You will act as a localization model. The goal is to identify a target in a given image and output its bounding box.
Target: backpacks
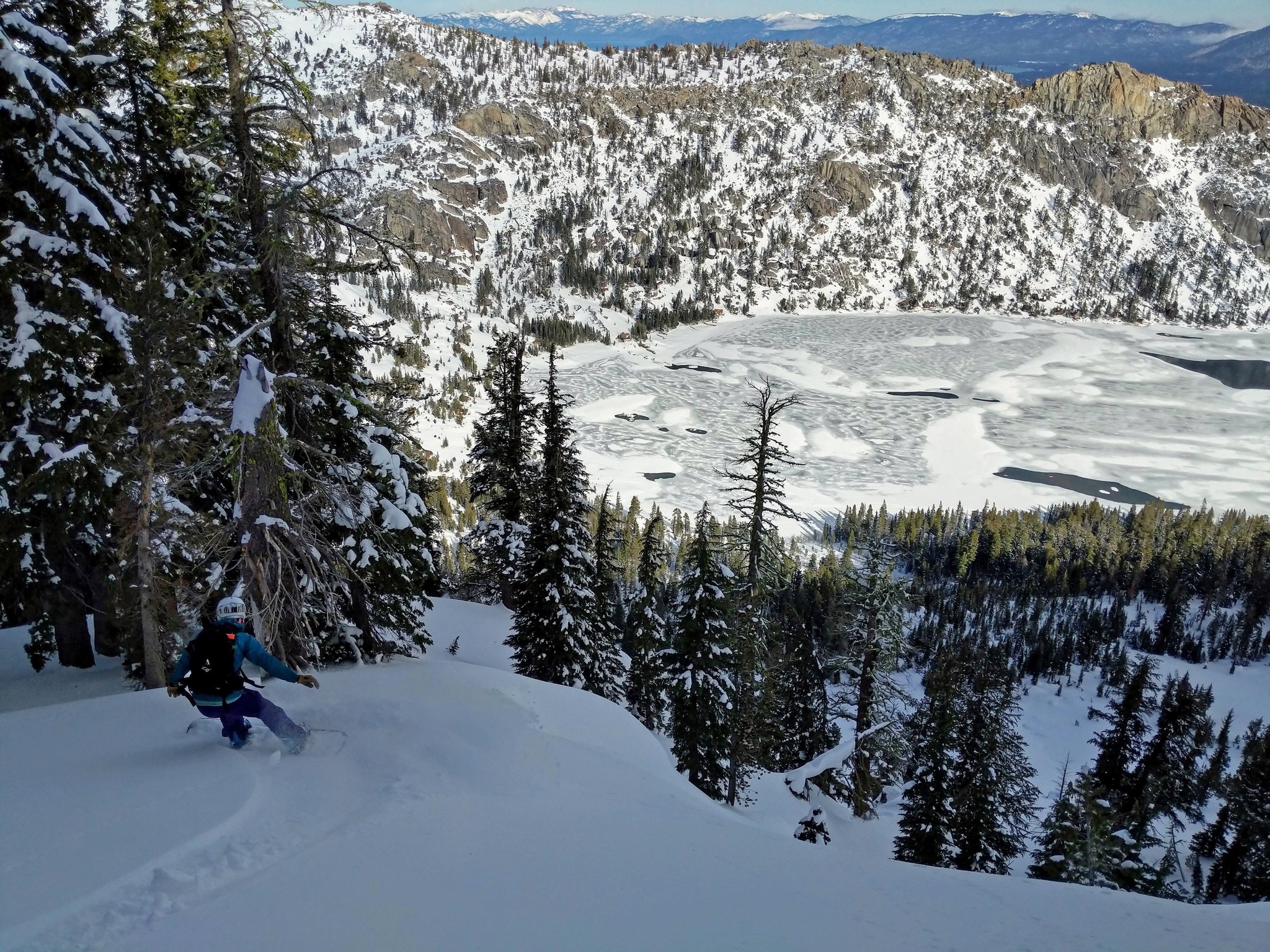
[188,624,241,697]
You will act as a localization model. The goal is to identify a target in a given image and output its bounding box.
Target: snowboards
[186,718,348,758]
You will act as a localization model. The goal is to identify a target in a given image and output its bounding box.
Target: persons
[167,598,319,754]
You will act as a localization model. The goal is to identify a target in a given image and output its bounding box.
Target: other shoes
[231,719,251,748]
[288,723,311,754]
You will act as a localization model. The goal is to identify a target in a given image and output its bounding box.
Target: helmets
[217,597,246,620]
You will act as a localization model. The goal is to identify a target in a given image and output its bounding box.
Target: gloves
[298,674,319,689]
[166,685,180,698]
